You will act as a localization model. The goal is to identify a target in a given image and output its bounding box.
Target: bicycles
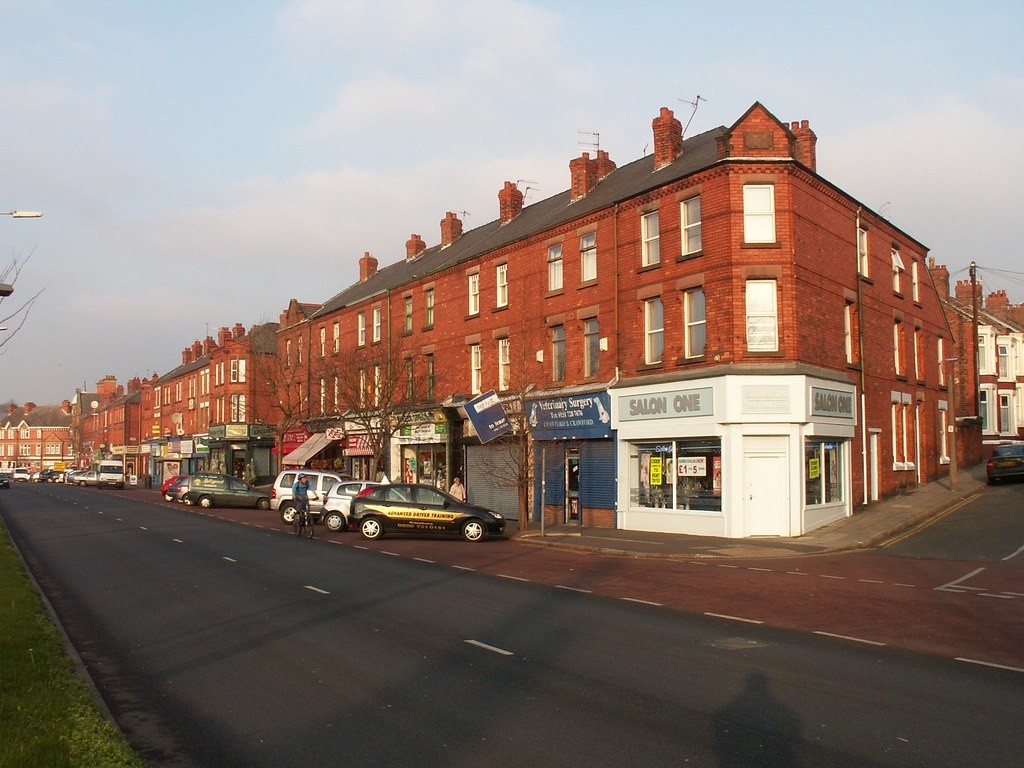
[293,496,316,539]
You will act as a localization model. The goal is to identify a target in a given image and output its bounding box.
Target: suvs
[67,470,86,485]
[270,469,358,526]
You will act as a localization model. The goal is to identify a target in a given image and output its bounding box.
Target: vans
[97,460,124,490]
[14,468,30,482]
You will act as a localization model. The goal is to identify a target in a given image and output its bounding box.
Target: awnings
[282,432,338,466]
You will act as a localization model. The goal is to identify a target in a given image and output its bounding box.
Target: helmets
[298,473,306,479]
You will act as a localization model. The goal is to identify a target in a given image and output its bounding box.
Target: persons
[292,473,319,529]
[448,477,467,503]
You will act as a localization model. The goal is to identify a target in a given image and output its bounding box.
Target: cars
[321,481,410,533]
[187,471,270,510]
[69,471,97,487]
[32,469,76,483]
[347,483,504,541]
[161,474,189,502]
[167,478,195,506]
[986,442,1024,486]
[0,474,10,489]
